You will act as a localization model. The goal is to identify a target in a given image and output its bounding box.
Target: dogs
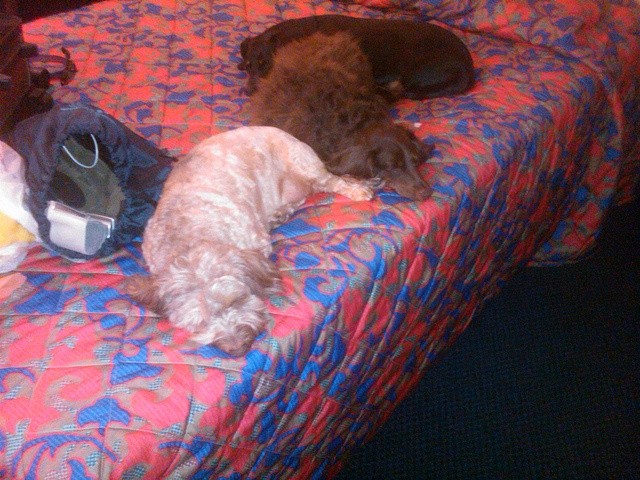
[124,126,376,357]
[250,30,435,200]
[238,14,473,100]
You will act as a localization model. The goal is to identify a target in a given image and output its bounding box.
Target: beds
[2,0,638,480]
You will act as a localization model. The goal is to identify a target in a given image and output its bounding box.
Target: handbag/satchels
[0,103,173,261]
